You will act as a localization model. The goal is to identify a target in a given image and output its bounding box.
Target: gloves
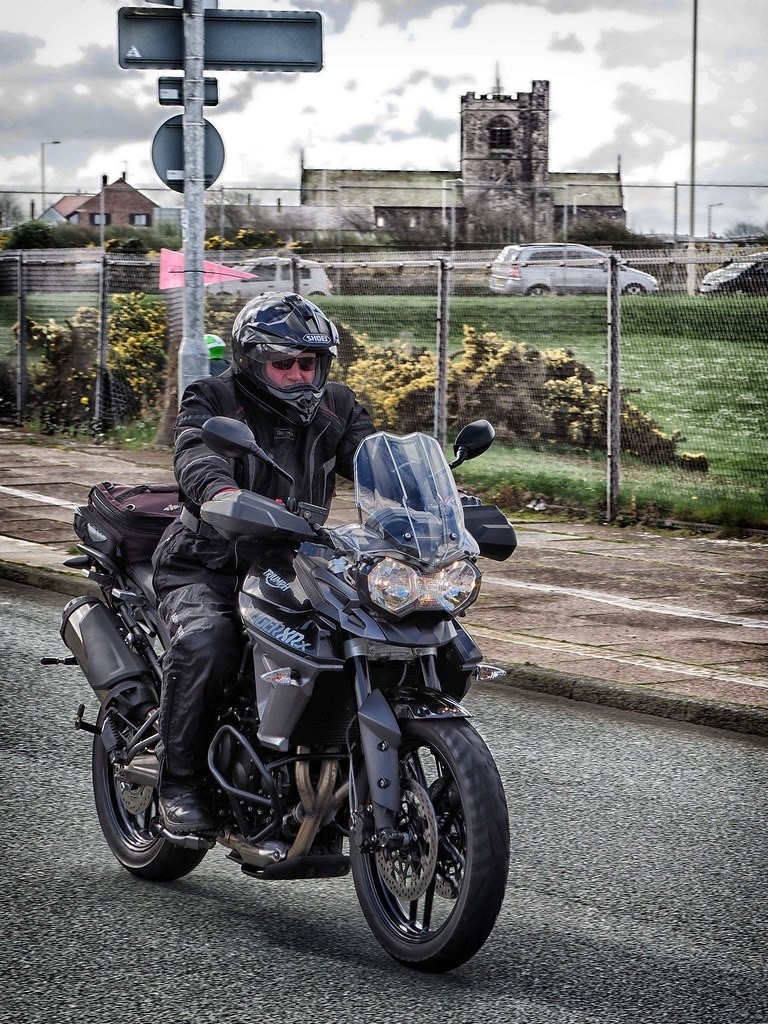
[444,492,483,508]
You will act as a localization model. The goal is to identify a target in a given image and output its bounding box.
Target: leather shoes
[158,792,215,832]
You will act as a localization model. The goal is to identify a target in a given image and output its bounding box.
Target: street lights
[37,138,63,213]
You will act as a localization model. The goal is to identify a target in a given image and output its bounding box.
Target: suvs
[488,241,660,296]
[204,257,337,299]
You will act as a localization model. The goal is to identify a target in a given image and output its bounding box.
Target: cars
[701,251,768,293]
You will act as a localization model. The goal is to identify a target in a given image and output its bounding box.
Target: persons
[150,291,484,833]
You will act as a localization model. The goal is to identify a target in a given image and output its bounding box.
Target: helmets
[231,291,339,427]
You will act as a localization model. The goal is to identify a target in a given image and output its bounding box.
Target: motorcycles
[40,419,519,961]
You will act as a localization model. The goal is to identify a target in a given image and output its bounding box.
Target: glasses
[263,351,319,371]
